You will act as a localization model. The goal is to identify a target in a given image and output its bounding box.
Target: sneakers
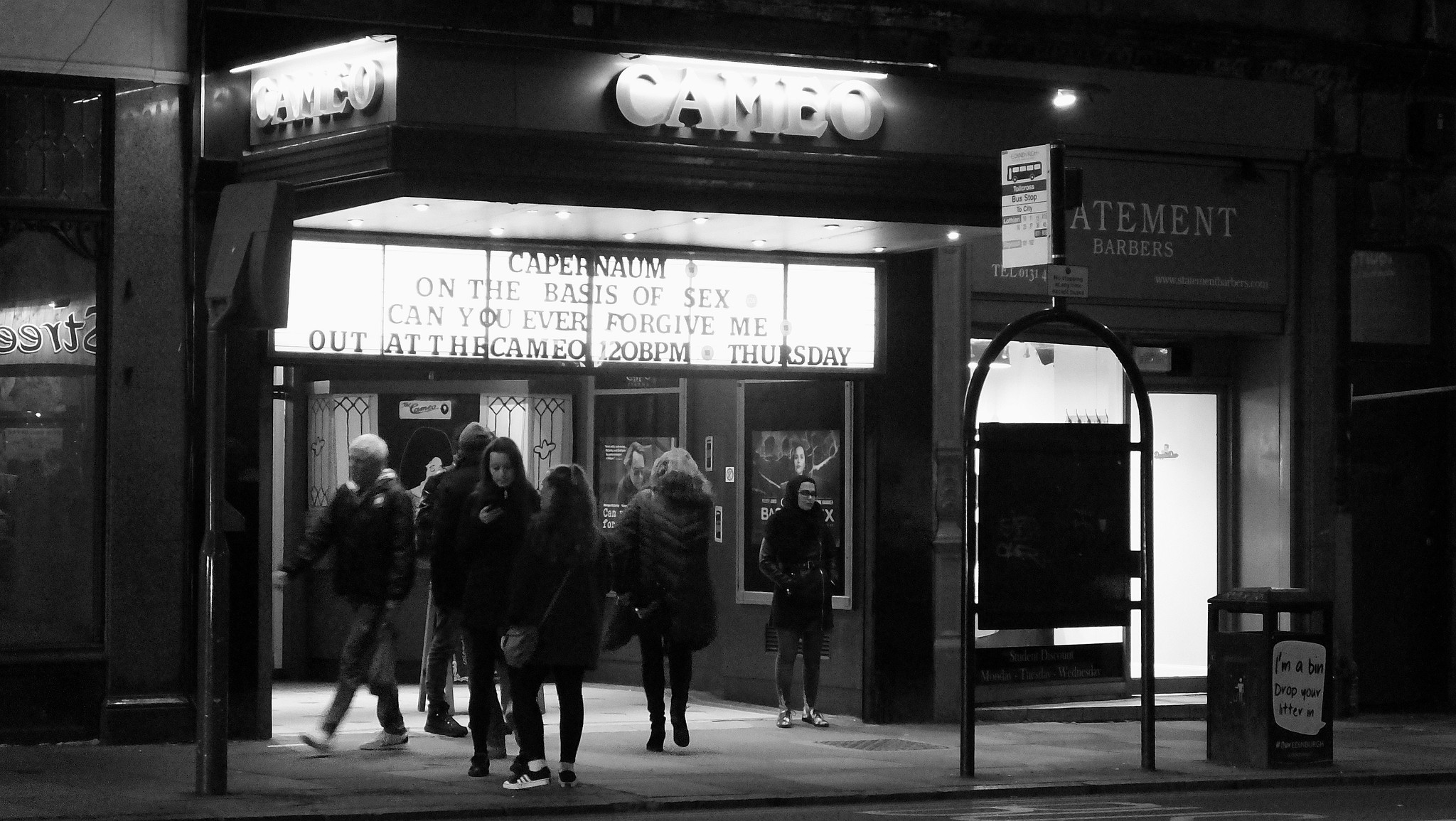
[360,729,410,750]
[558,768,577,787]
[302,729,338,755]
[424,704,468,738]
[802,708,829,728]
[776,710,792,729]
[503,766,551,789]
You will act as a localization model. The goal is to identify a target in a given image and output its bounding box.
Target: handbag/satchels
[499,625,539,667]
[599,591,639,650]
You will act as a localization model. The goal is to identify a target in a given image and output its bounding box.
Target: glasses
[798,490,818,498]
[631,466,646,475]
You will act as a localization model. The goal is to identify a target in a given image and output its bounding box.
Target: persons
[454,437,542,776]
[275,434,418,755]
[759,474,837,729]
[609,450,717,752]
[616,440,652,510]
[790,444,806,476]
[418,422,514,739]
[504,461,609,790]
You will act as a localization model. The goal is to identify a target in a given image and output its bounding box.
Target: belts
[778,560,830,571]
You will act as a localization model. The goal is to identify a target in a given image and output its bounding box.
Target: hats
[458,421,491,452]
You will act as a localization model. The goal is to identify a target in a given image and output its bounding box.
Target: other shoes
[468,755,490,777]
[510,750,533,773]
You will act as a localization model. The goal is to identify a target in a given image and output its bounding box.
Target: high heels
[671,707,690,747]
[646,725,666,752]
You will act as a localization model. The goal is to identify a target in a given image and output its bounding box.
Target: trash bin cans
[1205,586,1334,771]
